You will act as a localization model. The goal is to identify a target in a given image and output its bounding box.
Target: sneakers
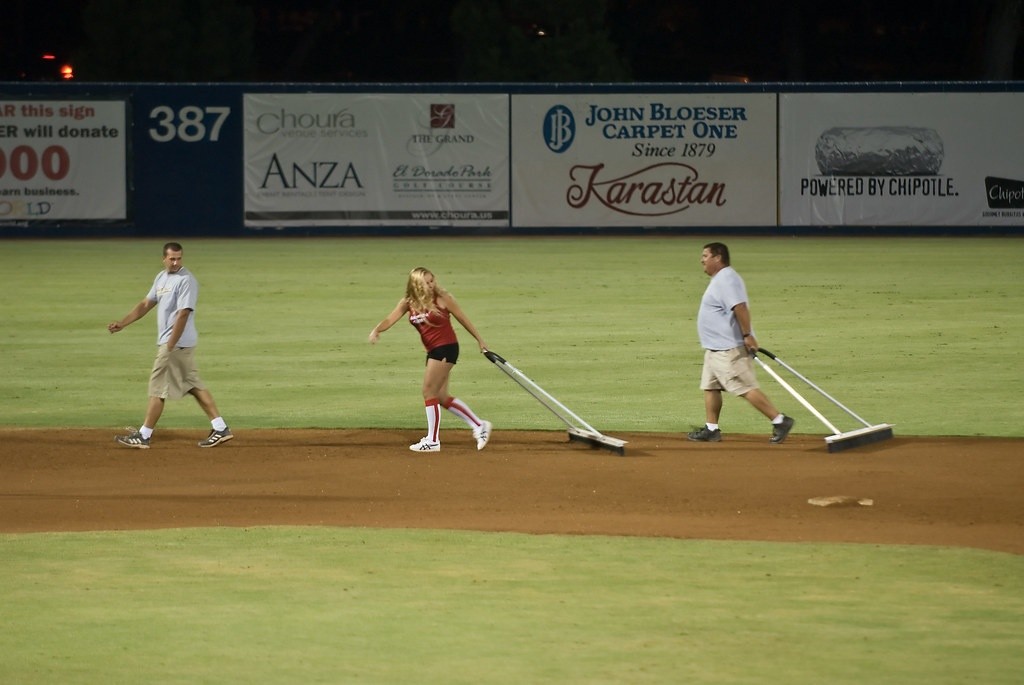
[410,436,440,452]
[473,421,492,451]
[117,426,151,449]
[198,426,233,447]
[687,424,722,442]
[769,413,794,444]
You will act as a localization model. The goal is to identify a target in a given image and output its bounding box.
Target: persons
[369,268,492,453]
[108,243,234,448]
[687,242,794,443]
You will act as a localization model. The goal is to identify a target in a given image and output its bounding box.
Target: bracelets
[743,333,751,339]
